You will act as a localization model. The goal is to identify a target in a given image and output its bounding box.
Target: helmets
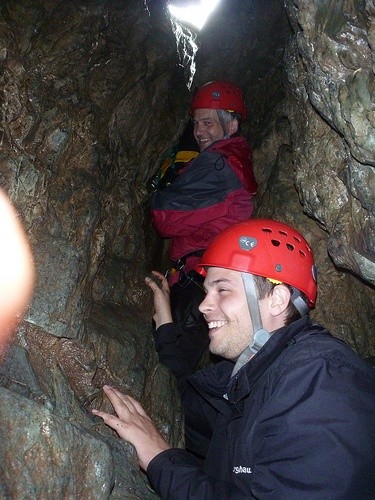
[191,80,248,119]
[194,216,320,307]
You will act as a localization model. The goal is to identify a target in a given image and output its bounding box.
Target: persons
[145,78,261,465]
[91,219,375,500]
